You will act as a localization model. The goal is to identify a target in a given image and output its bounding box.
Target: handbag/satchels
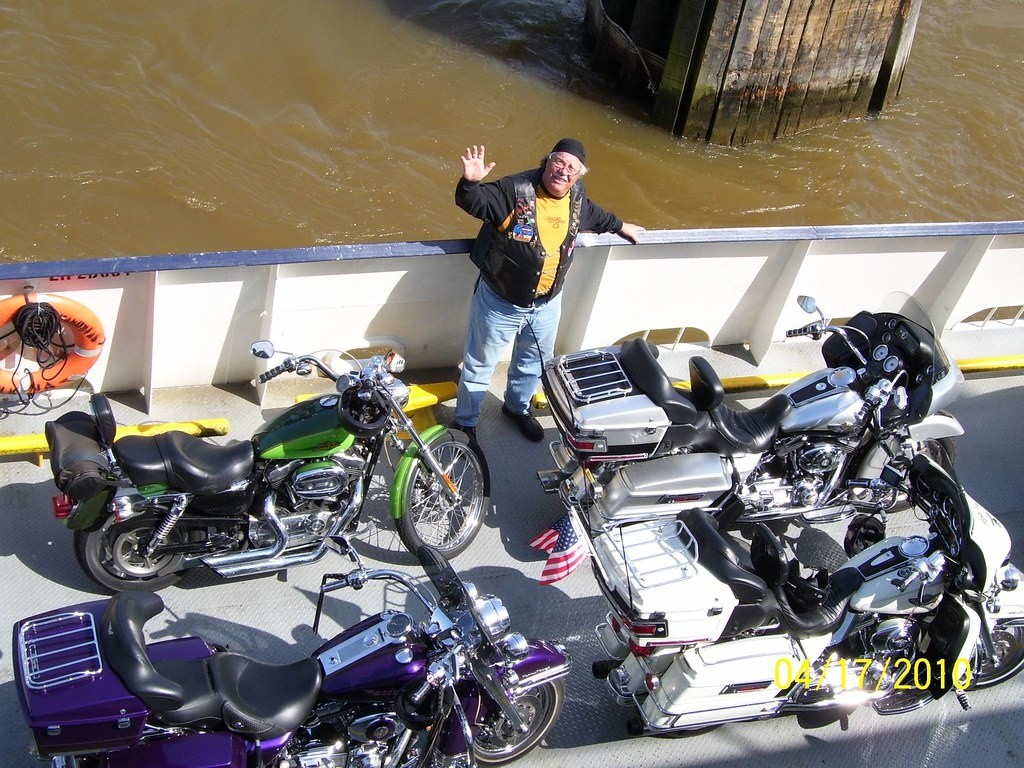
[45,411,110,499]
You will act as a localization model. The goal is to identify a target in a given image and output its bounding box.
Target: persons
[454,138,646,442]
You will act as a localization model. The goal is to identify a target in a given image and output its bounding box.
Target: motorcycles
[45,340,492,595]
[520,289,966,540]
[9,532,576,768]
[581,438,1024,738]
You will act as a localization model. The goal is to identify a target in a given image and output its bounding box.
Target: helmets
[338,380,392,438]
[393,675,454,733]
[844,514,886,558]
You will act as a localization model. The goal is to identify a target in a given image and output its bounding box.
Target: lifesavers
[0,290,107,394]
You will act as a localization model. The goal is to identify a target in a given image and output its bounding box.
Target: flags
[527,511,592,586]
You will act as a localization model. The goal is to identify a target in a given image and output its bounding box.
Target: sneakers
[453,422,476,459]
[502,402,544,442]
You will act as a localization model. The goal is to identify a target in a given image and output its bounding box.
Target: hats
[551,138,585,165]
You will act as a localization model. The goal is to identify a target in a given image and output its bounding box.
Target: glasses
[548,156,580,176]
